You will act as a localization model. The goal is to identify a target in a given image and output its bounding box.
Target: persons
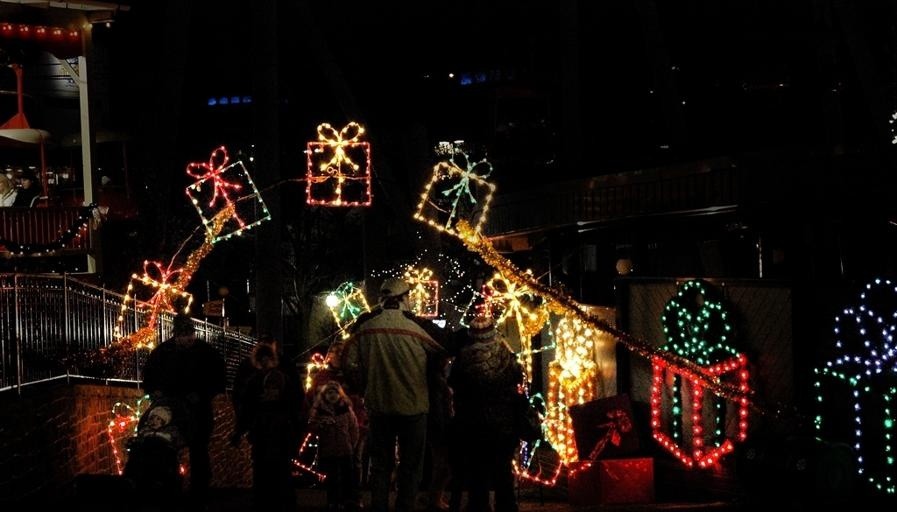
[13,169,39,209]
[121,268,542,510]
[0,174,18,209]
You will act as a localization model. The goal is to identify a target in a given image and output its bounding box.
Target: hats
[169,313,200,334]
[148,404,174,427]
[379,276,412,298]
[467,316,495,340]
[321,379,344,399]
